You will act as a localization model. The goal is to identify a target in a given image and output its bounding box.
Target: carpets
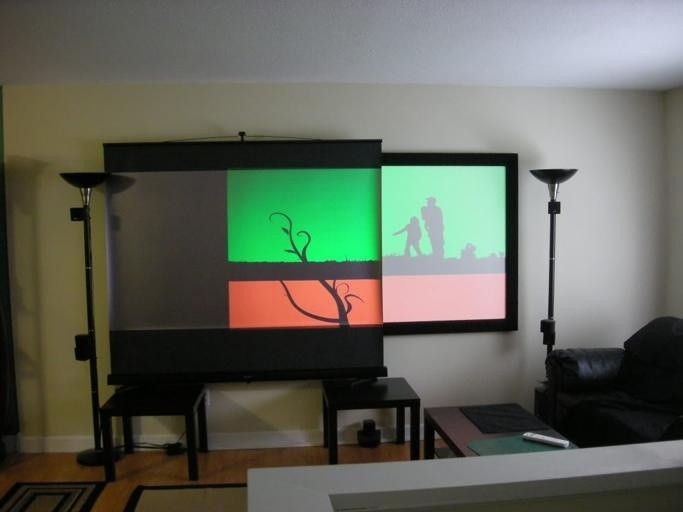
[0,478,246,512]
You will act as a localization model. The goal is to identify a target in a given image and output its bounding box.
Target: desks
[94,379,211,484]
[321,375,420,466]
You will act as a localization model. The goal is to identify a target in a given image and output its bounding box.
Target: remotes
[522,432,569,448]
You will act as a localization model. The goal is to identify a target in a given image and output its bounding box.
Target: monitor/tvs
[217,152,519,336]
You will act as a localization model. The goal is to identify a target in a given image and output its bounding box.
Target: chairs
[532,316,681,443]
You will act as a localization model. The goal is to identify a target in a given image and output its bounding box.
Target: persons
[393,216,421,256]
[421,197,444,257]
